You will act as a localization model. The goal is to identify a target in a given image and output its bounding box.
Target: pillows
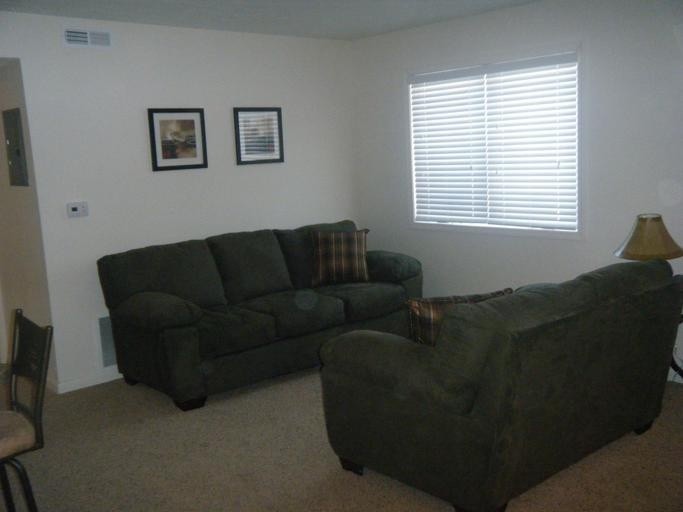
[396,288,512,347]
[311,228,369,288]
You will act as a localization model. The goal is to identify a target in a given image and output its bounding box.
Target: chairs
[0,309,53,511]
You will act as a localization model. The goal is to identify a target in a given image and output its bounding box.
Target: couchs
[320,262,682,511]
[95,219,422,410]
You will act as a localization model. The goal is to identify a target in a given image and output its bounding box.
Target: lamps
[613,213,682,261]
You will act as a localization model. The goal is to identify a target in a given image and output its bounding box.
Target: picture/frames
[147,108,208,171]
[233,107,284,166]
